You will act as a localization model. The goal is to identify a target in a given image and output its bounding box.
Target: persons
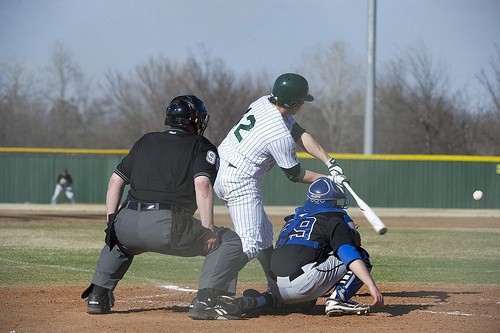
[50,168,76,207]
[80,94,245,321]
[211,73,353,306]
[235,176,385,319]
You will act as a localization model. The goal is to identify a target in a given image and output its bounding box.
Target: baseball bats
[343,180,387,235]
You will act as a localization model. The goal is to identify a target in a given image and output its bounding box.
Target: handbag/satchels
[171,203,211,250]
[104,213,117,252]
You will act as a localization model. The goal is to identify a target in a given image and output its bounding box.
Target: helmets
[270,73,314,110]
[164,94,210,136]
[307,177,349,209]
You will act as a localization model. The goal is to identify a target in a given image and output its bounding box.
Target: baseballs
[472,189,483,200]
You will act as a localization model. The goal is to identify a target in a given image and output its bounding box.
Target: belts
[122,199,171,211]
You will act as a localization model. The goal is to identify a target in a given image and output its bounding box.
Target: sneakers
[188,297,244,320]
[324,298,370,317]
[217,295,257,314]
[86,292,110,313]
